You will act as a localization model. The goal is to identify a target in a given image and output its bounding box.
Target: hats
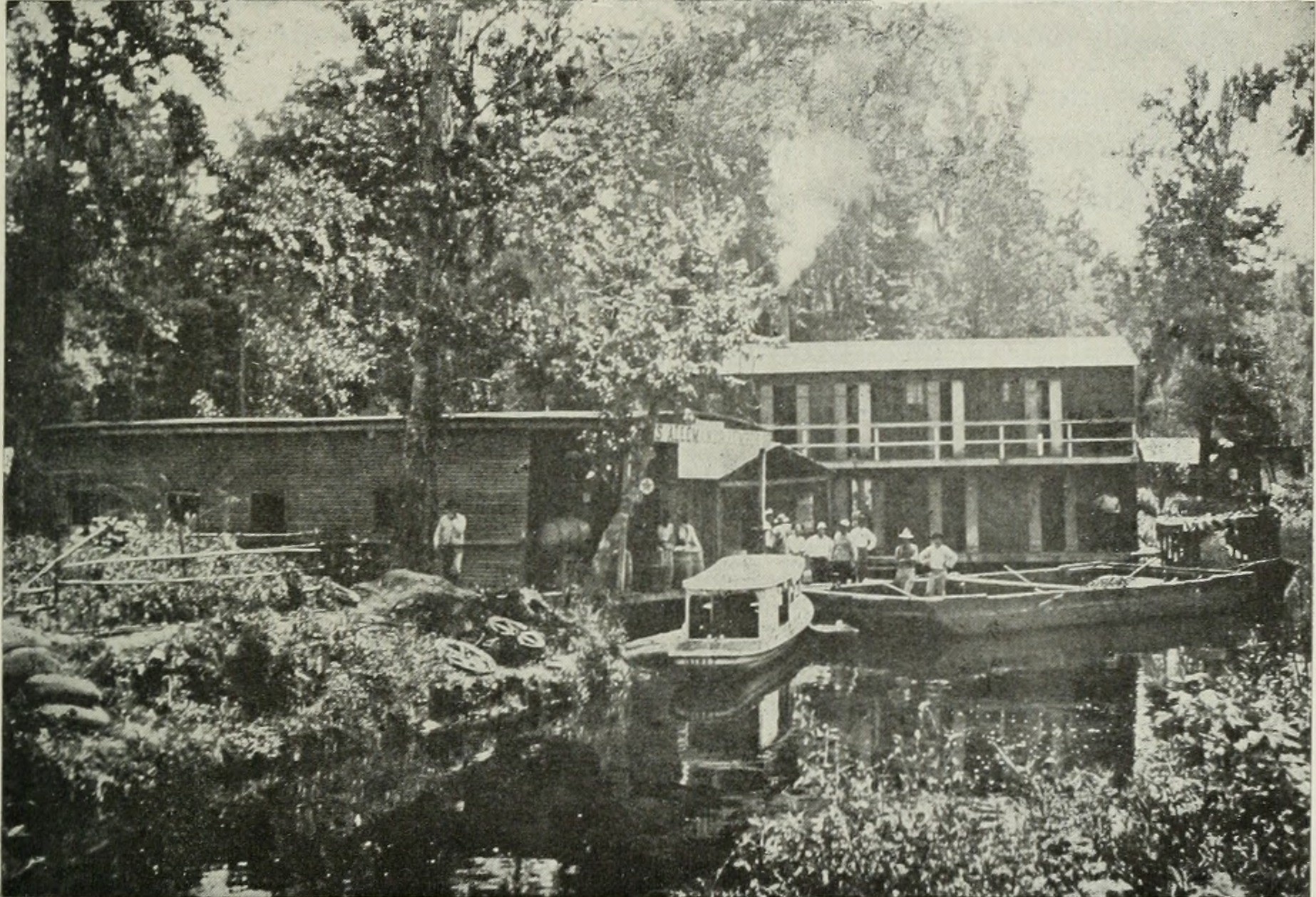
[817,521,827,530]
[898,526,914,539]
[444,499,460,509]
[774,513,789,524]
[840,518,850,527]
[927,531,942,539]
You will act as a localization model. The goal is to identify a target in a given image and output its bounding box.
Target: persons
[764,508,958,596]
[1092,486,1122,550]
[657,511,704,580]
[432,498,468,585]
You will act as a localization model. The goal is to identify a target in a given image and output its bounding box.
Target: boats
[616,551,816,684]
[794,554,1260,638]
